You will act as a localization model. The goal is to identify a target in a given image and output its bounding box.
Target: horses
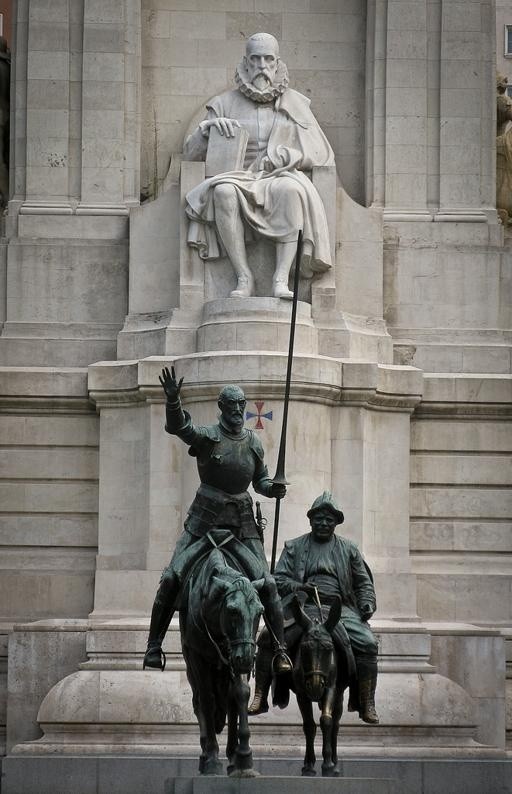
[289,593,347,777]
[180,550,266,778]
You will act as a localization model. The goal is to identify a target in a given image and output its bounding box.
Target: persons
[140,360,295,676]
[180,31,332,304]
[247,490,386,731]
[490,71,511,230]
